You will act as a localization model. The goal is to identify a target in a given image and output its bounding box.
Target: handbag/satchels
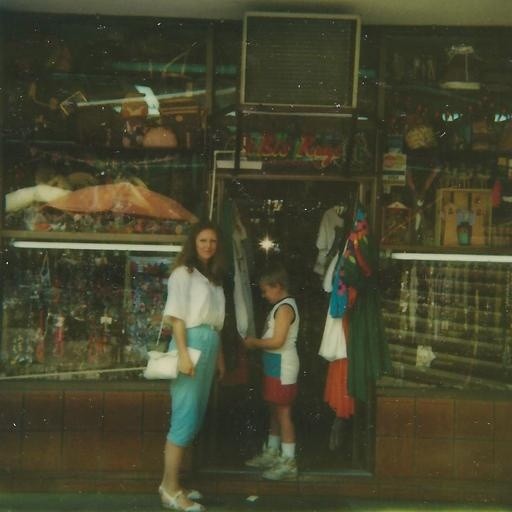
[142,349,180,381]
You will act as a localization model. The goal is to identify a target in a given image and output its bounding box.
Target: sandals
[161,490,208,511]
[159,484,204,501]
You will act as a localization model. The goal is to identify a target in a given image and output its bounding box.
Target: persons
[243,262,299,481]
[156,217,227,511]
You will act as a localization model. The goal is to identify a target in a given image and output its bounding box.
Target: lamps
[144,116,177,149]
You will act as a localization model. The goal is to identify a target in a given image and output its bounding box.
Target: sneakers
[262,456,299,481]
[244,442,280,468]
